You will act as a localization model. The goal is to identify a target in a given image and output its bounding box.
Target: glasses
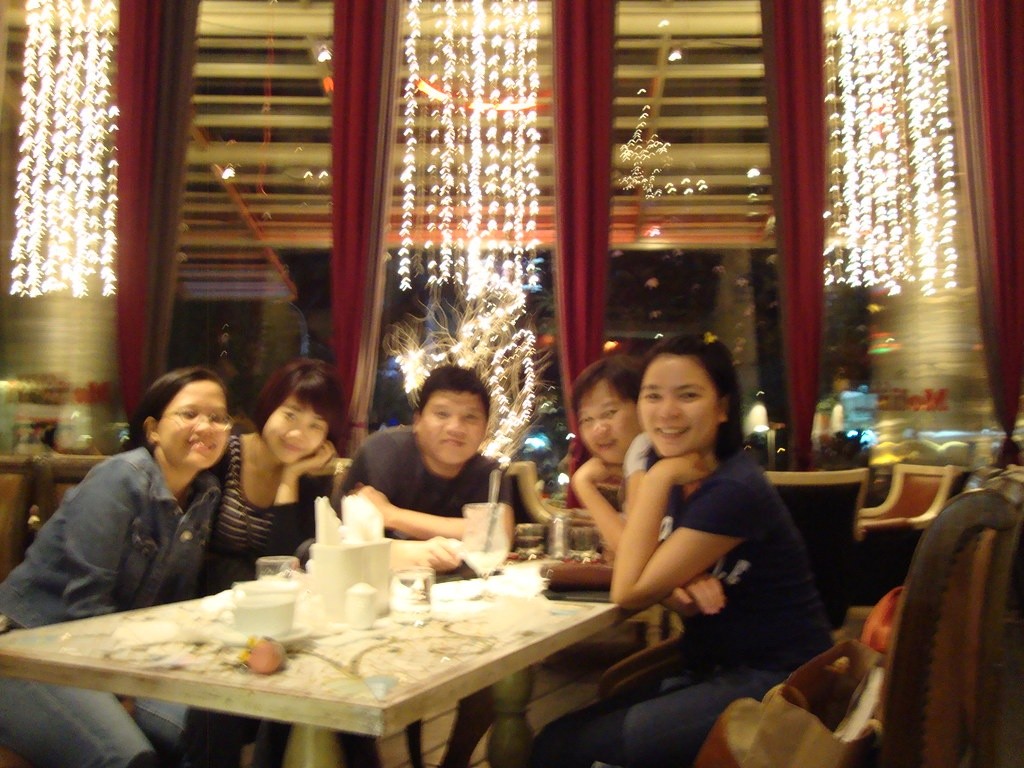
[159,407,236,431]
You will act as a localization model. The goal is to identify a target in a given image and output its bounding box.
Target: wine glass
[460,501,517,603]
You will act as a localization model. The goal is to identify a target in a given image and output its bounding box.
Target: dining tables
[0,554,659,768]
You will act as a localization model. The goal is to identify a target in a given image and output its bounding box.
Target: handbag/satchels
[859,583,905,655]
[699,635,890,768]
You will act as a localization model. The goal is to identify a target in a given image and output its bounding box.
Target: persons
[534,333,833,768]
[334,366,515,768]
[0,362,340,768]
[570,357,640,554]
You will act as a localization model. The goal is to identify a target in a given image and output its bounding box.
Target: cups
[517,522,545,560]
[254,555,301,597]
[229,596,299,638]
[563,518,599,565]
[389,565,436,627]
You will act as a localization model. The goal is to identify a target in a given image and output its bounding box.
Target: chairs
[0,451,1024,768]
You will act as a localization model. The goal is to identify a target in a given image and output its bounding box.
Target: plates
[210,625,312,650]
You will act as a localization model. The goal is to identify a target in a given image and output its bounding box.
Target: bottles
[346,582,379,631]
